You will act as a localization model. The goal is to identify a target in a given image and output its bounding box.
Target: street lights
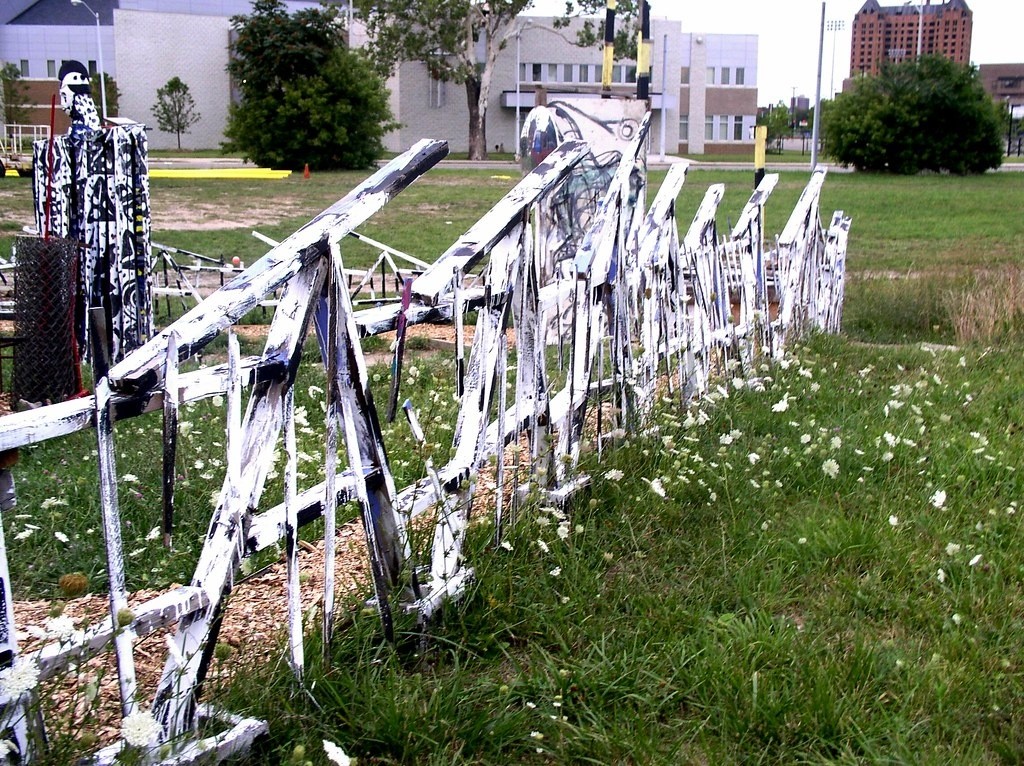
[515,18,533,162]
[70,0,108,134]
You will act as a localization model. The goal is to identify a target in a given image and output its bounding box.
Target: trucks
[0,123,53,177]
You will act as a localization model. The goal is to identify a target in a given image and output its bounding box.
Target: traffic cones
[303,163,311,179]
